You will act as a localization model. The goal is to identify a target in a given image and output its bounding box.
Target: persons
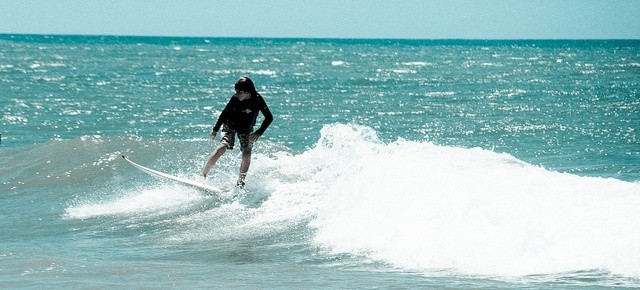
[201,76,273,188]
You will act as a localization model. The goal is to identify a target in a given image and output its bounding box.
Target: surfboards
[121,153,223,201]
